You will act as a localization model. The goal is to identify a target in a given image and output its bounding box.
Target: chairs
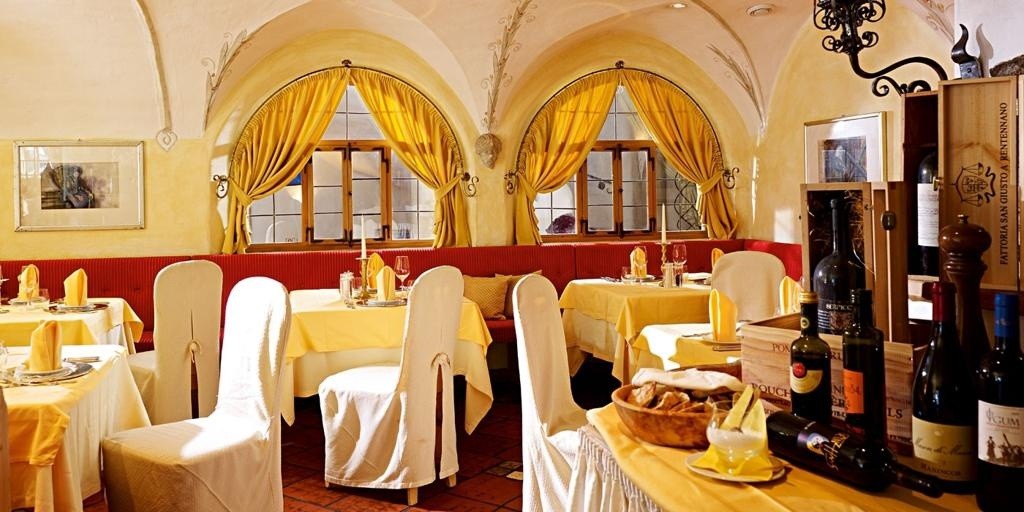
[711,251,785,327]
[97,274,290,512]
[127,260,221,424]
[266,222,298,242]
[318,263,465,505]
[512,273,587,512]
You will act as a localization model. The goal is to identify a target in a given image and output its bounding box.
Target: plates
[3,364,94,383]
[9,297,43,304]
[46,302,107,315]
[686,448,786,481]
[356,297,409,308]
[700,334,746,346]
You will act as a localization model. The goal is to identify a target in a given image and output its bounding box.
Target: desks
[281,289,495,435]
[0,296,145,354]
[0,344,152,512]
[575,397,1024,512]
[558,271,710,384]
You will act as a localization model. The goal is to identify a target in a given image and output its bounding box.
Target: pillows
[461,274,511,319]
[495,270,542,319]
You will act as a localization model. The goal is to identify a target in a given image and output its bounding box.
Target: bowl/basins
[609,382,728,449]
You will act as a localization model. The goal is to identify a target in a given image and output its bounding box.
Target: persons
[63,166,93,208]
[986,433,1024,466]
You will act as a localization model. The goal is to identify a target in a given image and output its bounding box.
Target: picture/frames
[13,140,143,232]
[804,112,887,184]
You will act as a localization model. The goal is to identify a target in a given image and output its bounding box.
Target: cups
[37,289,53,305]
[672,244,688,266]
[621,266,637,284]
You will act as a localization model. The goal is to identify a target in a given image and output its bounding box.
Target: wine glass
[633,245,649,281]
[394,255,411,296]
[19,265,41,311]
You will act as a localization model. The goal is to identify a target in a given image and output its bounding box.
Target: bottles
[909,139,939,277]
[981,289,1023,511]
[675,267,684,286]
[339,271,358,300]
[763,408,942,499]
[787,288,834,425]
[908,277,979,490]
[840,286,891,434]
[811,198,866,334]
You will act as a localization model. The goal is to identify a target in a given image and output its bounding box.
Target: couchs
[13,238,804,391]
